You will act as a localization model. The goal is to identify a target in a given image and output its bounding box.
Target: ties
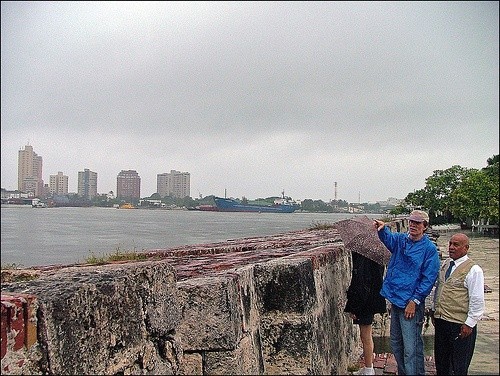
[445,261,455,281]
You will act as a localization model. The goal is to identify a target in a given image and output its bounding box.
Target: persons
[430,233,484,376]
[344,252,387,376]
[373,210,439,376]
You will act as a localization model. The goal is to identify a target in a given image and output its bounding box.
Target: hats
[406,210,429,223]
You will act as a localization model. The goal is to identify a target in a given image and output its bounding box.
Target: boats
[121,203,136,209]
[213,196,296,213]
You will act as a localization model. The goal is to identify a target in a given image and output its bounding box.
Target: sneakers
[353,363,375,375]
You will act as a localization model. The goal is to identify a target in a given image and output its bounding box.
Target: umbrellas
[333,214,392,267]
